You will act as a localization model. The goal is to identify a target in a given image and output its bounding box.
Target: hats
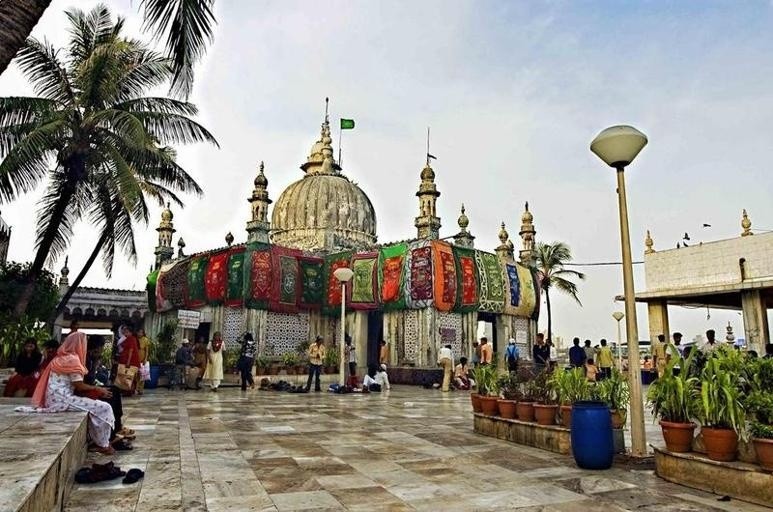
[180,339,189,343]
[509,338,515,343]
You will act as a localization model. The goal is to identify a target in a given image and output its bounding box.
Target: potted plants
[199,343,355,375]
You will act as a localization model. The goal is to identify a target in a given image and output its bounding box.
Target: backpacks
[507,345,516,367]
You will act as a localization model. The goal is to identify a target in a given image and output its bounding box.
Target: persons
[176,339,206,370]
[533,333,558,371]
[437,337,492,393]
[202,332,226,392]
[569,338,615,382]
[237,331,255,390]
[653,330,772,380]
[644,356,651,368]
[345,336,356,376]
[363,364,391,392]
[73,333,134,445]
[3,321,82,398]
[380,340,387,364]
[14,331,116,456]
[304,336,326,391]
[504,339,519,374]
[117,326,149,397]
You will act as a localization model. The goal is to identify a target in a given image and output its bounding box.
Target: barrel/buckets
[570,400,614,470]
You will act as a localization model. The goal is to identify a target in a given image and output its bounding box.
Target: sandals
[76,427,143,485]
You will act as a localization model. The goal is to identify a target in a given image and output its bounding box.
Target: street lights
[610,310,624,375]
[588,123,649,457]
[332,267,353,389]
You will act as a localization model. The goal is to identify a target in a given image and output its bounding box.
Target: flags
[340,118,354,129]
